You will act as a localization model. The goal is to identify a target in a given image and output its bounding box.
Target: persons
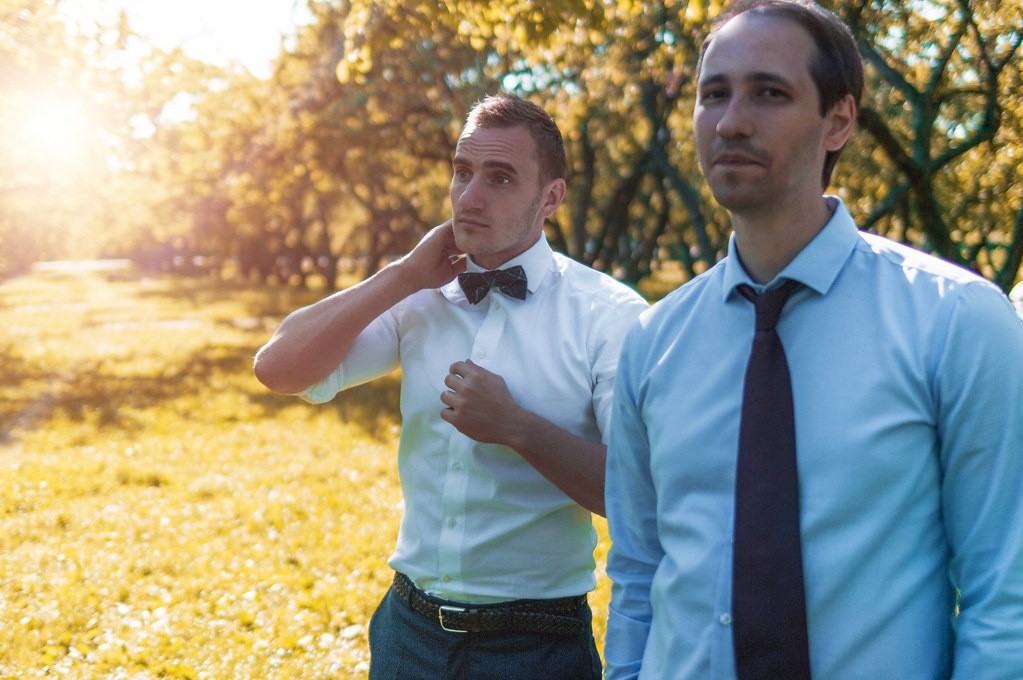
[605,0,1023,680]
[252,91,649,680]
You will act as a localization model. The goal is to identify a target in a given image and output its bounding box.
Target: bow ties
[457,265,528,304]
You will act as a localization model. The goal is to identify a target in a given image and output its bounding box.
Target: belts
[392,571,587,637]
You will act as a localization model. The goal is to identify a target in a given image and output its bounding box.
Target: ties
[737,279,812,680]
